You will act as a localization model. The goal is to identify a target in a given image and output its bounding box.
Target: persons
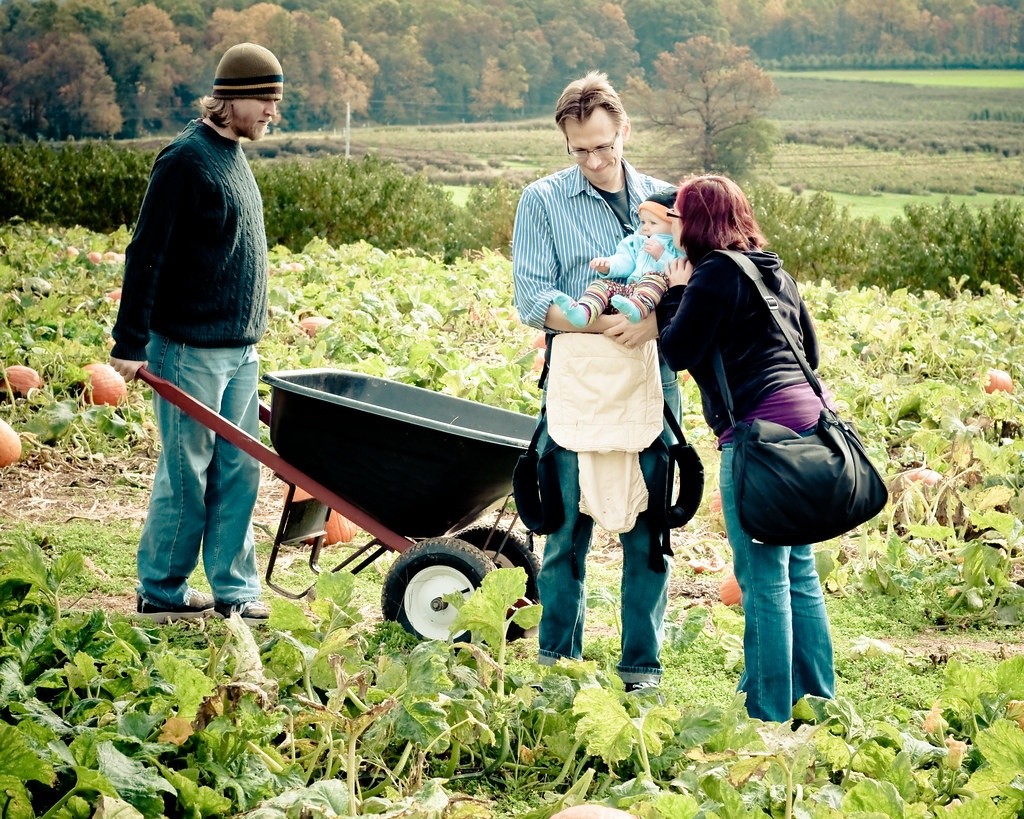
[550,186,683,329]
[655,174,834,731]
[109,44,286,619]
[511,76,685,692]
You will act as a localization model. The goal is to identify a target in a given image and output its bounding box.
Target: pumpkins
[0,245,1016,609]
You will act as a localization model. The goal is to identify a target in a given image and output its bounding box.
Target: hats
[212,42,284,100]
[638,185,679,225]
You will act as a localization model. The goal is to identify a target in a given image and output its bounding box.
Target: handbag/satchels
[733,408,889,546]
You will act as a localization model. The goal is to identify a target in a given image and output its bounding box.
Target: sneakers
[625,681,666,707]
[135,587,271,620]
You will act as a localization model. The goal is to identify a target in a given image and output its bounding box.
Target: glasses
[566,128,622,159]
[666,209,684,220]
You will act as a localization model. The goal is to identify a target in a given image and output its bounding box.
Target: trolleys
[135,362,546,654]
